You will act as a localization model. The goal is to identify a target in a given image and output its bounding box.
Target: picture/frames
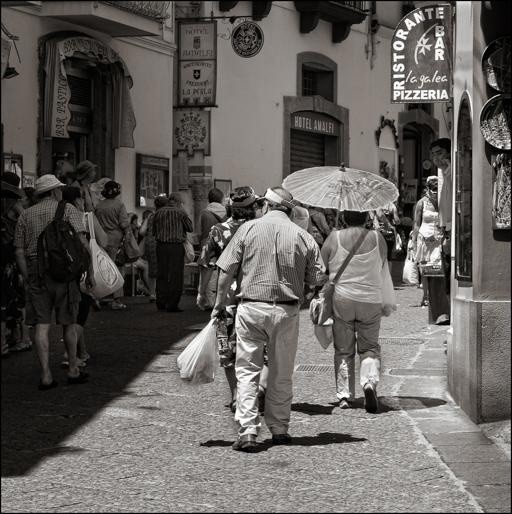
[214,178,233,198]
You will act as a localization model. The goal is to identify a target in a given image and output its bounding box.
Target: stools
[125,261,140,296]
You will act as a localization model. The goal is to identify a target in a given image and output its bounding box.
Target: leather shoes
[232,434,256,451]
[272,433,292,444]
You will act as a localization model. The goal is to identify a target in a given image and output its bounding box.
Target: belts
[241,298,298,305]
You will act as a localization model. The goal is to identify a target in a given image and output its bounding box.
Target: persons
[414,175,446,307]
[195,185,269,416]
[431,138,451,227]
[319,209,389,414]
[13,173,97,390]
[407,229,414,259]
[291,199,347,248]
[61,186,108,367]
[55,157,227,314]
[364,201,399,268]
[211,186,327,449]
[0,171,33,356]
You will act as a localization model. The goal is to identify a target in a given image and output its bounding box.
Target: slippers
[39,350,90,389]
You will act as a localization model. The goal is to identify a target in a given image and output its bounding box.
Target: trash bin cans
[423,272,450,325]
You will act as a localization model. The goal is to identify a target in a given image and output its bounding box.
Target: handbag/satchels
[403,259,420,285]
[37,218,89,284]
[80,238,124,301]
[115,231,142,267]
[309,282,335,325]
[184,240,195,263]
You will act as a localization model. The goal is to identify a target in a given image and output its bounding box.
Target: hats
[76,160,98,181]
[257,187,295,209]
[34,175,67,195]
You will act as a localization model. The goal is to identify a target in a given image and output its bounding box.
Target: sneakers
[339,398,351,408]
[111,302,127,310]
[364,384,379,413]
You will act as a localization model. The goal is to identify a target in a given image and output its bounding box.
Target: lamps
[2,33,22,79]
